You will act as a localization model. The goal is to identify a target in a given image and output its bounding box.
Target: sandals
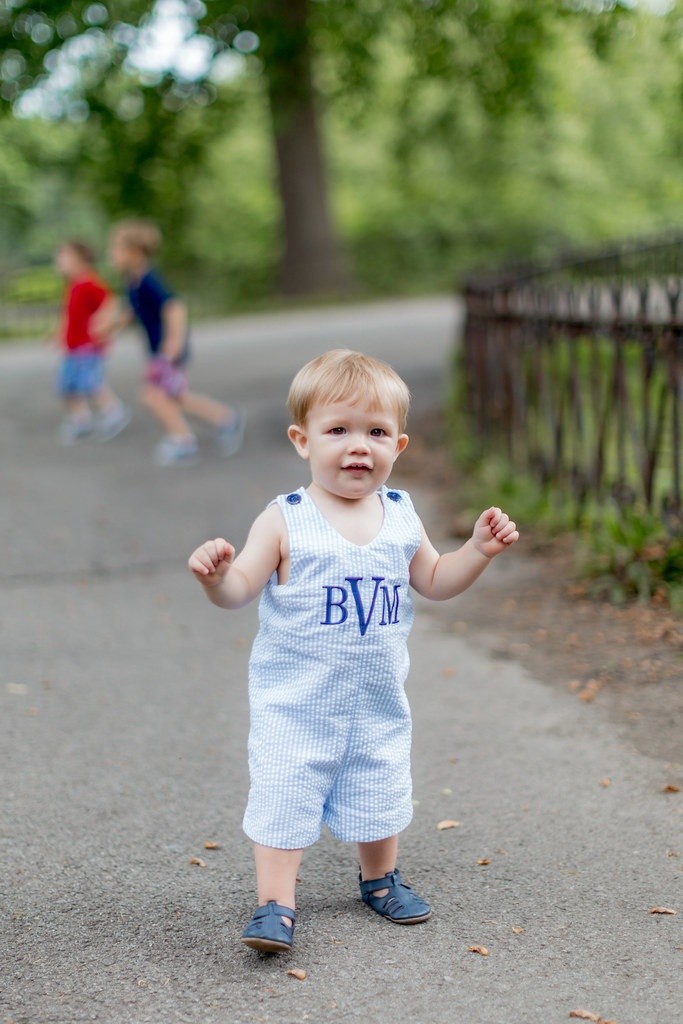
[242,901,296,952]
[357,863,431,923]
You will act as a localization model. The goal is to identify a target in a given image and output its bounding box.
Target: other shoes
[99,404,132,443]
[219,407,247,459]
[59,416,99,441]
[159,433,201,466]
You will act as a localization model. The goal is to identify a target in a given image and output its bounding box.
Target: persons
[189,350,519,951]
[49,218,246,463]
